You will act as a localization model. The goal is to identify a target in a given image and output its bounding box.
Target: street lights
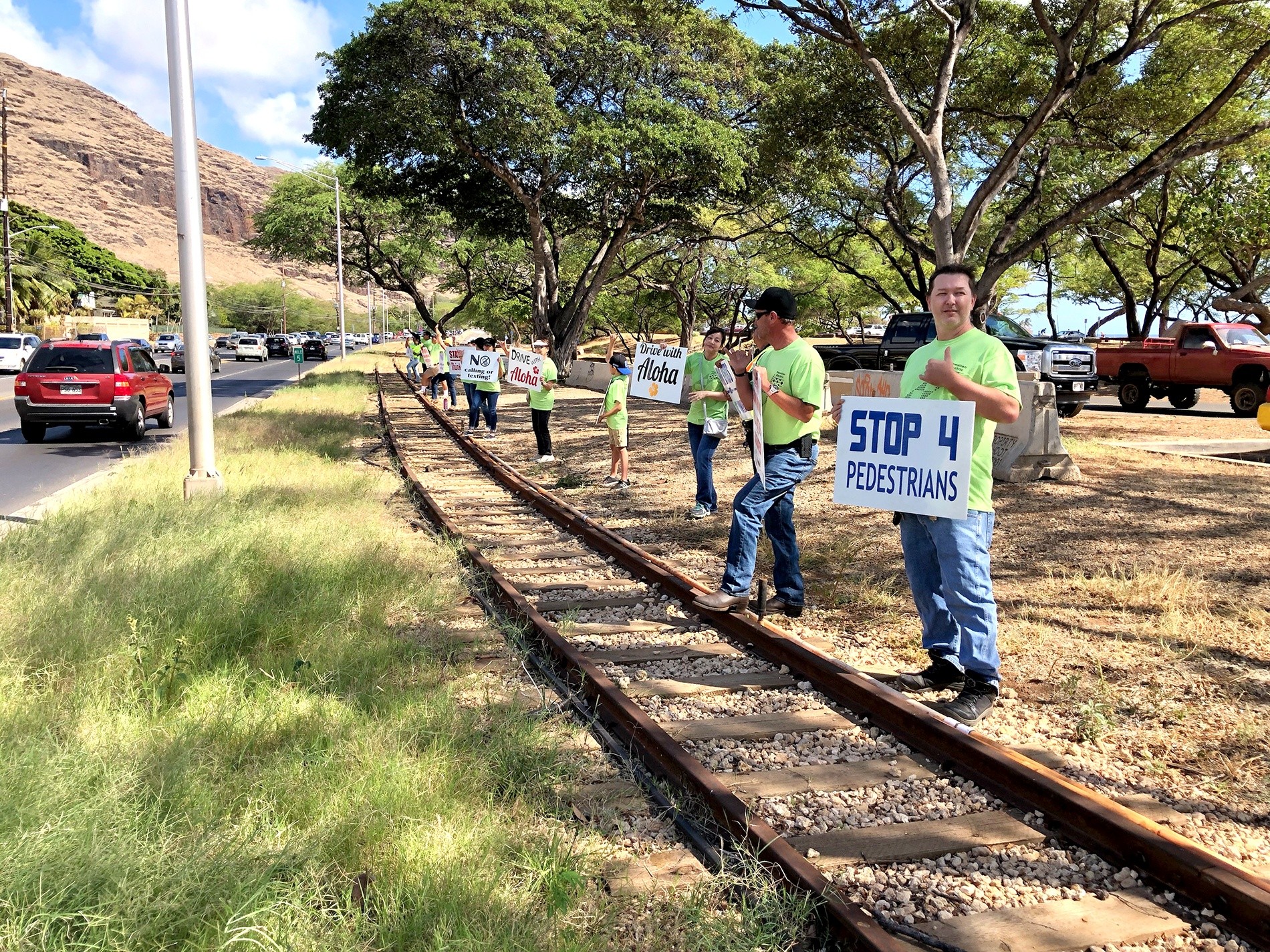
[7,215,60,333]
[345,278,372,349]
[398,299,411,329]
[256,154,346,359]
[283,291,297,332]
[372,287,385,344]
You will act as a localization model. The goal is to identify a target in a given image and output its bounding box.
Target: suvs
[836,324,888,336]
[13,338,174,442]
[0,333,42,373]
[153,333,182,353]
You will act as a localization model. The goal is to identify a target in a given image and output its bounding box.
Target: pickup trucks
[813,312,1099,418]
[1096,322,1270,417]
[1257,385,1270,430]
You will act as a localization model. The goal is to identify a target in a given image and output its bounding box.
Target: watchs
[767,383,780,396]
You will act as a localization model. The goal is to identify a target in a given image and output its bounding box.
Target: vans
[382,332,397,339]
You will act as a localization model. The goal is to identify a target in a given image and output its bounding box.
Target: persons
[715,318,772,540]
[1041,328,1046,334]
[693,286,825,618]
[830,264,1023,727]
[415,336,449,398]
[405,335,422,384]
[1101,332,1105,337]
[497,337,558,463]
[430,328,457,411]
[660,326,732,519]
[595,333,632,490]
[420,331,434,392]
[1038,331,1040,336]
[452,335,505,439]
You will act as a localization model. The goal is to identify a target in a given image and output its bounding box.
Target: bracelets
[734,371,747,377]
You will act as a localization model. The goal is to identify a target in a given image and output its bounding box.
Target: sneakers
[690,507,718,514]
[613,479,631,490]
[690,500,711,518]
[942,677,995,725]
[602,476,619,486]
[897,660,967,692]
[463,430,473,436]
[482,434,496,440]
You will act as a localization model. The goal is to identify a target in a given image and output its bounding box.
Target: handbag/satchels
[702,418,728,439]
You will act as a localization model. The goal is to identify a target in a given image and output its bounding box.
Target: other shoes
[448,405,456,412]
[407,377,427,395]
[430,399,439,404]
[441,395,449,399]
[535,454,554,463]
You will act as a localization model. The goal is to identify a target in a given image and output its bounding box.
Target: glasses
[534,346,547,349]
[755,311,770,320]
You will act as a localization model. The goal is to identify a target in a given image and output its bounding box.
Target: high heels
[692,589,748,614]
[749,597,802,618]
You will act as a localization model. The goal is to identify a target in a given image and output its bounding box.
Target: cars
[216,337,229,348]
[208,334,217,348]
[325,332,357,350]
[171,345,221,374]
[431,330,462,337]
[356,332,381,346]
[122,338,153,358]
[695,327,708,335]
[228,331,329,363]
[722,324,753,334]
[396,329,422,338]
[1049,331,1084,343]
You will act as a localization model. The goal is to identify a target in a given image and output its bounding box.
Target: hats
[485,338,495,346]
[610,354,632,375]
[444,337,452,347]
[531,340,548,346]
[744,287,798,320]
